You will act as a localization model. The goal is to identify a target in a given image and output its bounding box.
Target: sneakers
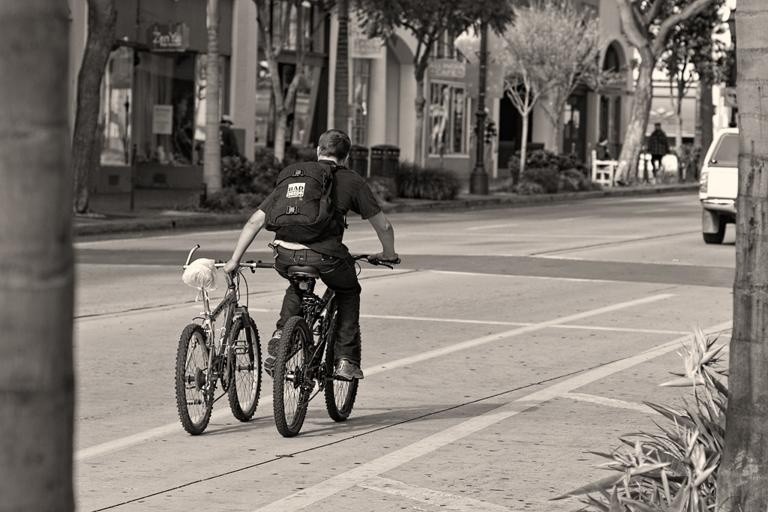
[333,359,364,382]
[264,334,281,378]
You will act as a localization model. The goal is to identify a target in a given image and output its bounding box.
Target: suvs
[698,128,745,249]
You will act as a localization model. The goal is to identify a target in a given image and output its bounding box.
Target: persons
[220,113,239,157]
[646,122,670,172]
[219,128,397,383]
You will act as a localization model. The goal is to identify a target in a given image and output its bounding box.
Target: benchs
[588,146,619,188]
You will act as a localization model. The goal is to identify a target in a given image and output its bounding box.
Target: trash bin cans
[370,145,400,186]
[349,146,368,178]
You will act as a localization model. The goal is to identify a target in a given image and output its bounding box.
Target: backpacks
[264,158,351,231]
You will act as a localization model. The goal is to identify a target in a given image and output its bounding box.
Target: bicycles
[173,241,266,435]
[242,251,406,436]
